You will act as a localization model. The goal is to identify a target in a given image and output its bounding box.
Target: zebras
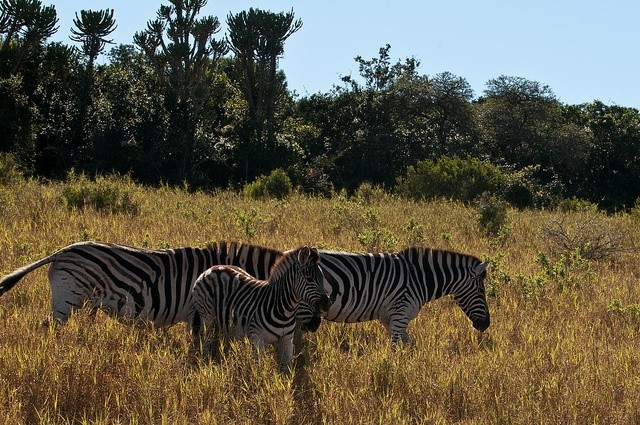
[282,246,490,353]
[187,245,333,374]
[0,241,321,366]
